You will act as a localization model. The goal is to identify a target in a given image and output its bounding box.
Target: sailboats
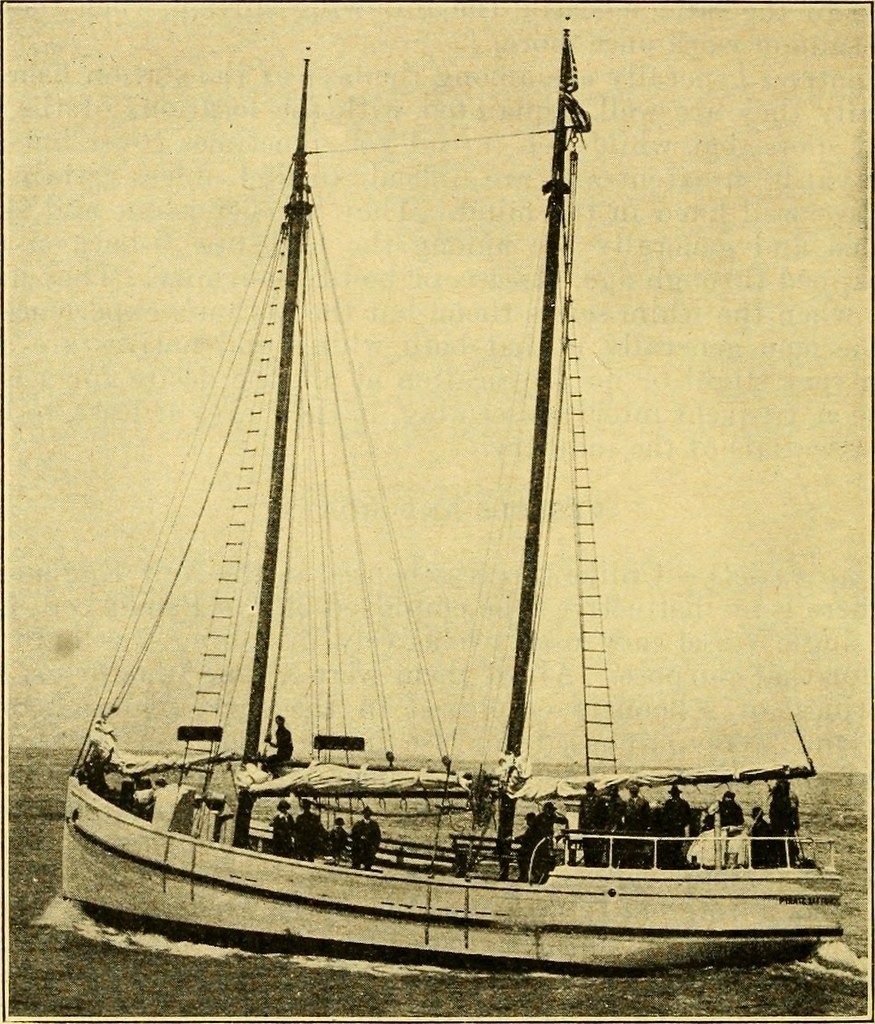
[60,17,845,978]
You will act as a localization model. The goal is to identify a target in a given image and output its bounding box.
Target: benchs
[449,832,555,884]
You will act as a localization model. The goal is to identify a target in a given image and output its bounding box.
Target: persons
[88,715,381,870]
[504,781,800,884]
